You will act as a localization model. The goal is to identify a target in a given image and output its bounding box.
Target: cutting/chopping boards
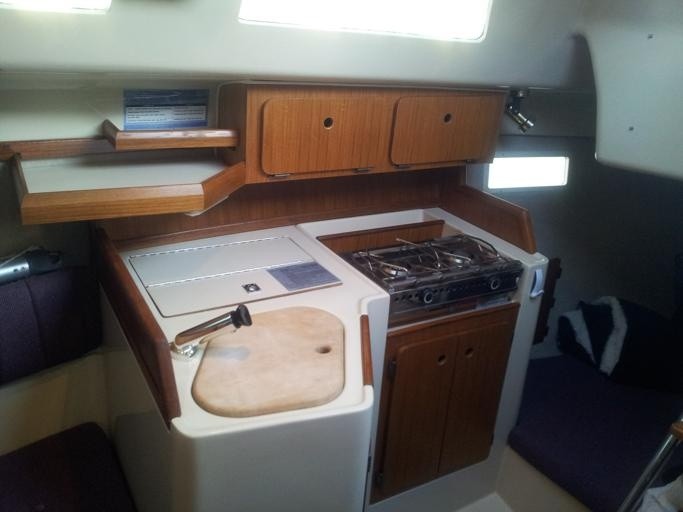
[191,305,348,419]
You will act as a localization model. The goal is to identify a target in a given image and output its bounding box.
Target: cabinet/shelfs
[220,88,508,183]
[370,301,520,504]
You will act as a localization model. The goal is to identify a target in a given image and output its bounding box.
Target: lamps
[502,89,539,134]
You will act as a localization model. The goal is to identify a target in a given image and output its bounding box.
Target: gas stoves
[341,235,522,328]
